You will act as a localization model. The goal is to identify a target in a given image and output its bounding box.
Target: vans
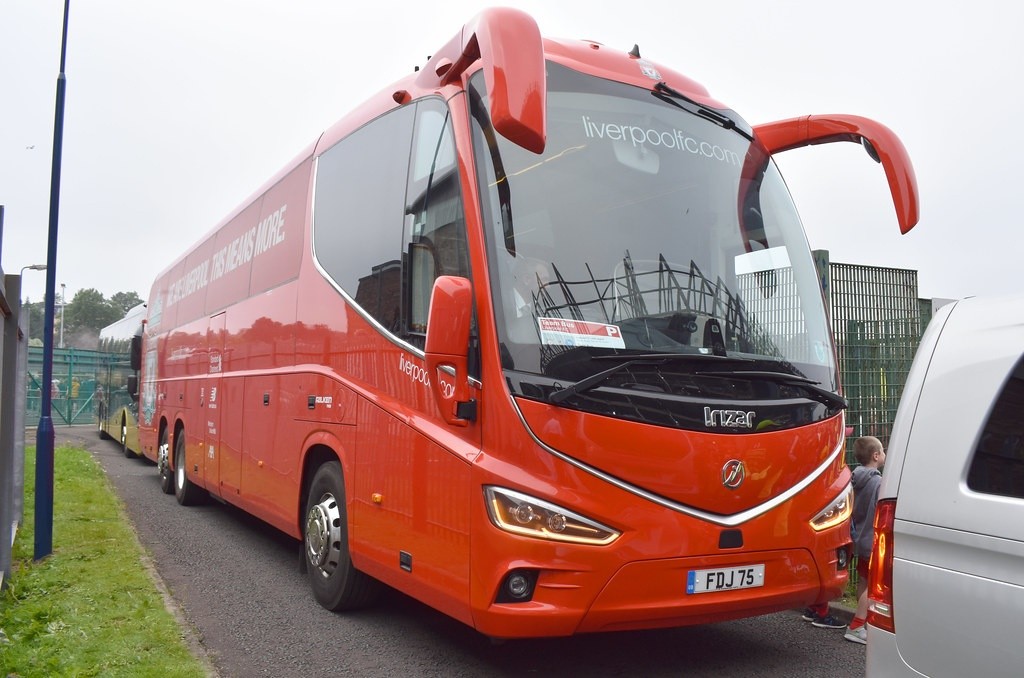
[863,296,1024,678]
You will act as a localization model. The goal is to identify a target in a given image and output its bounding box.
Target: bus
[96,301,149,459]
[144,3,923,646]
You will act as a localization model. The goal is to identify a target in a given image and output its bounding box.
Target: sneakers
[802,609,821,621]
[844,626,868,645]
[812,613,847,629]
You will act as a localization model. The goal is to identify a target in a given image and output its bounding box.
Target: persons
[802,427,854,628]
[511,257,551,318]
[66,376,81,411]
[844,435,886,645]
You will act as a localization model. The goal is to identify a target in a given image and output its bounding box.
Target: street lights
[59,282,67,349]
